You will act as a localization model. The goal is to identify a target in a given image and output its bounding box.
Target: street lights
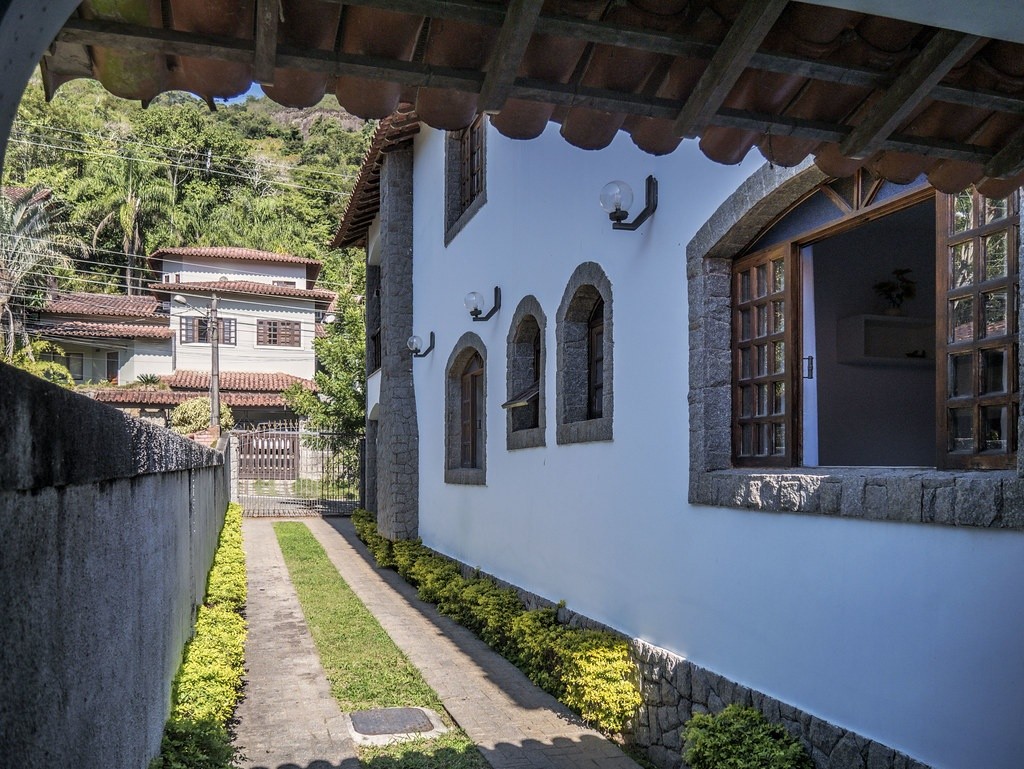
[173,287,221,431]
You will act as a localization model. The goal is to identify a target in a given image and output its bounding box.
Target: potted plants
[872,267,916,317]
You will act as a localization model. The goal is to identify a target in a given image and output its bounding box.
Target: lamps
[599,174,658,231]
[407,330,435,357]
[463,285,502,321]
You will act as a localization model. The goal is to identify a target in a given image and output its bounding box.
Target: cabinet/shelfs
[836,312,936,371]
